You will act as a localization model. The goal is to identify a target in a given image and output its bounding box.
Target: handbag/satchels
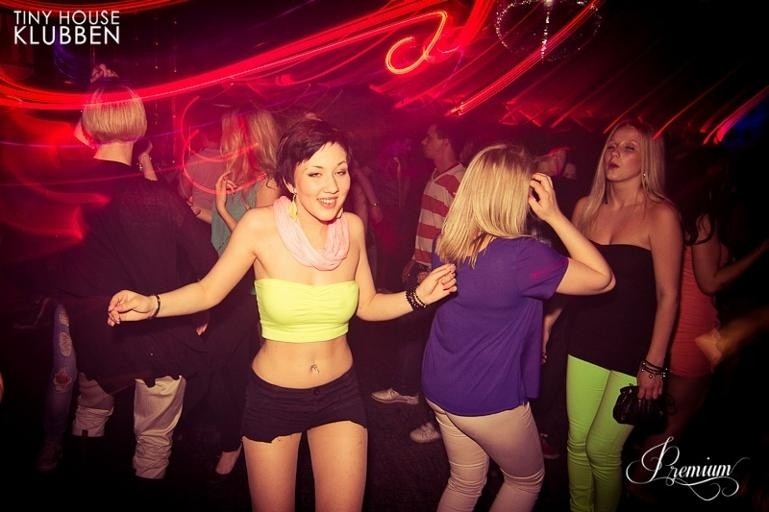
[610,384,672,434]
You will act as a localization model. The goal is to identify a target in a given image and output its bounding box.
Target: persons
[1,61,768,511]
